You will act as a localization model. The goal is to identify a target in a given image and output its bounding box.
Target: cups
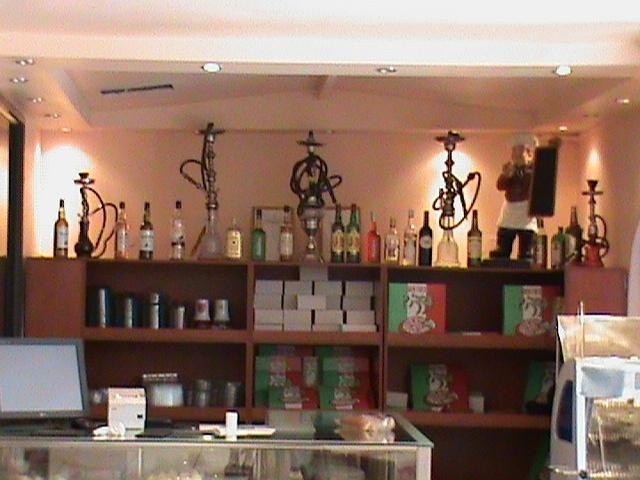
[194,296,212,322]
[213,300,230,322]
[169,305,186,329]
[115,296,140,329]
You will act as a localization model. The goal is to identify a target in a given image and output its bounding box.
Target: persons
[488,130,538,259]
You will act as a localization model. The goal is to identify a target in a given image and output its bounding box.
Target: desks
[547,354,640,479]
[0,411,435,480]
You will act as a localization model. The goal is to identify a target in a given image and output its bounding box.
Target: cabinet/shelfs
[385,268,565,423]
[251,264,384,413]
[76,262,254,413]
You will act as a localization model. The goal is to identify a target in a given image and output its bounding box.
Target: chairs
[553,311,640,476]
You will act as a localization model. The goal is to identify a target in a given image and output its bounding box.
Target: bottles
[467,210,483,269]
[96,287,111,328]
[187,377,212,406]
[251,208,266,261]
[330,202,344,263]
[114,202,129,258]
[54,199,69,258]
[224,216,243,258]
[198,200,222,259]
[367,211,381,265]
[139,201,155,260]
[279,205,293,260]
[346,203,360,264]
[436,209,459,266]
[534,204,584,270]
[418,210,433,265]
[384,216,400,266]
[169,200,187,260]
[148,292,160,330]
[402,208,417,266]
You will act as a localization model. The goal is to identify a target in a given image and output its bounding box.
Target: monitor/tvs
[0,337,90,436]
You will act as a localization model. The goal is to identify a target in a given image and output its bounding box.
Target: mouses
[70,417,90,429]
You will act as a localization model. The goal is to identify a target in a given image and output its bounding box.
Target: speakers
[527,147,557,217]
